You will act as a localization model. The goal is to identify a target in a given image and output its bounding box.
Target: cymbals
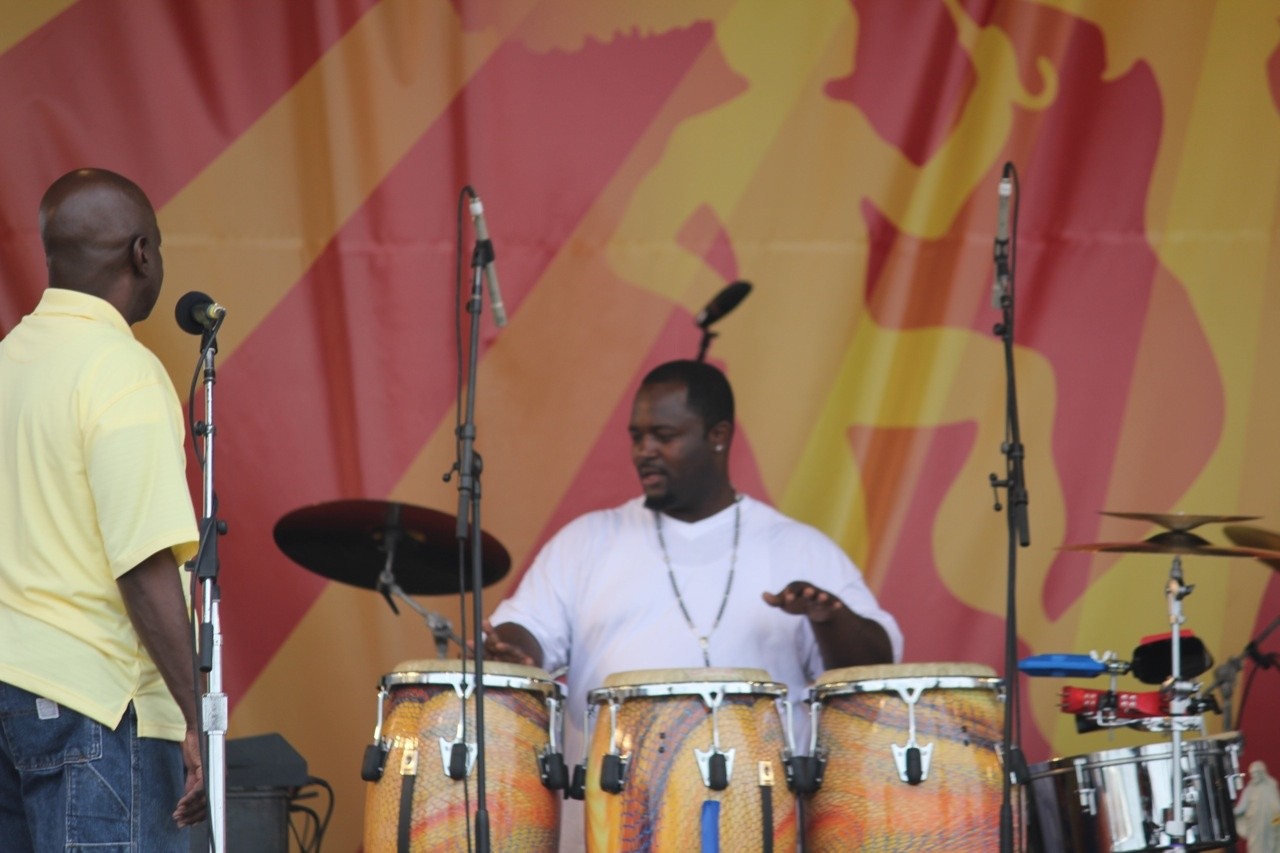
[273,498,511,597]
[1055,506,1279,561]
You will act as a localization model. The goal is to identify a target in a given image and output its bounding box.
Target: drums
[361,660,569,853]
[569,664,804,853]
[802,659,1027,853]
[1027,731,1246,853]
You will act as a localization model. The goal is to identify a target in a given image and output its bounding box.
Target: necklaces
[652,489,740,671]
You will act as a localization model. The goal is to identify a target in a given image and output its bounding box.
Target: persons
[0,168,209,853]
[461,359,906,853]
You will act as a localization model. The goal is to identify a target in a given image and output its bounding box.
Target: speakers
[190,791,289,853]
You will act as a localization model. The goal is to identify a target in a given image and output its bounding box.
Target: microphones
[469,188,506,325]
[695,282,752,327]
[175,291,225,334]
[992,174,1012,308]
[1018,653,1132,678]
